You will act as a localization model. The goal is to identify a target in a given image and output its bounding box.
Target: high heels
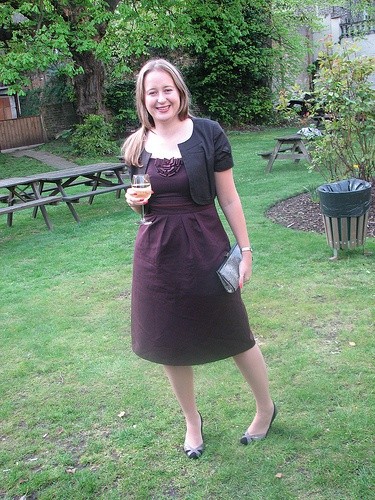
[240,401,277,445]
[183,411,205,460]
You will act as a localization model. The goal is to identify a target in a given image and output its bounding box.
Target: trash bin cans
[318,180,372,261]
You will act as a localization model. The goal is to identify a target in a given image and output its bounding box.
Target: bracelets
[240,247,252,254]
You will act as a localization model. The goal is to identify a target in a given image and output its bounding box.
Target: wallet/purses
[216,242,242,293]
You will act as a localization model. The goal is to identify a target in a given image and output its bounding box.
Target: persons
[120,59,278,460]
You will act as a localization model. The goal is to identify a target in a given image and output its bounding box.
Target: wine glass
[130,173,153,225]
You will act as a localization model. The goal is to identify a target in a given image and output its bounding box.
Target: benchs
[105,170,115,176]
[255,144,298,156]
[61,182,131,202]
[0,194,12,204]
[0,196,61,215]
[311,114,345,121]
[16,178,95,196]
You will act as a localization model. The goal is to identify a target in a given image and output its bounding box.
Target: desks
[266,135,321,172]
[25,162,132,224]
[0,176,53,232]
[288,100,308,117]
[115,154,131,200]
